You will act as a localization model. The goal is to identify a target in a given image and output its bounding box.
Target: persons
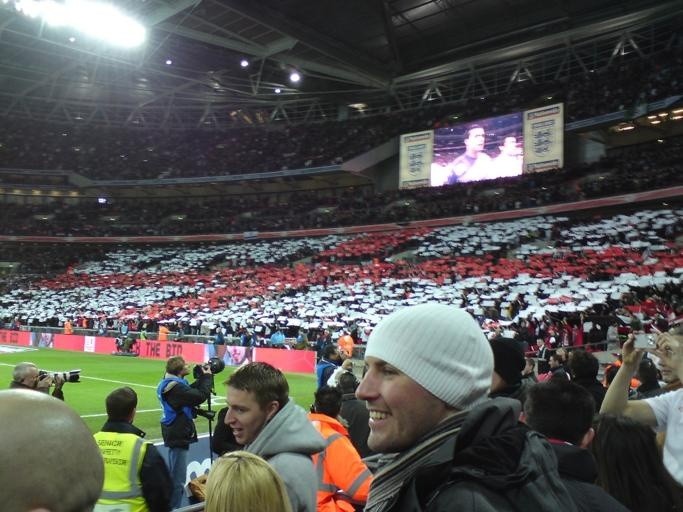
[1,44,682,512]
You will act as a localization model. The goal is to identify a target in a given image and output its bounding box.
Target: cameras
[194,356,225,380]
[36,365,81,385]
[189,407,218,420]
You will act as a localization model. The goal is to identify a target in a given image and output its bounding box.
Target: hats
[488,337,526,385]
[364,301,496,412]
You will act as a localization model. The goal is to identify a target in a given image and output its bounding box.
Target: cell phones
[631,331,660,351]
